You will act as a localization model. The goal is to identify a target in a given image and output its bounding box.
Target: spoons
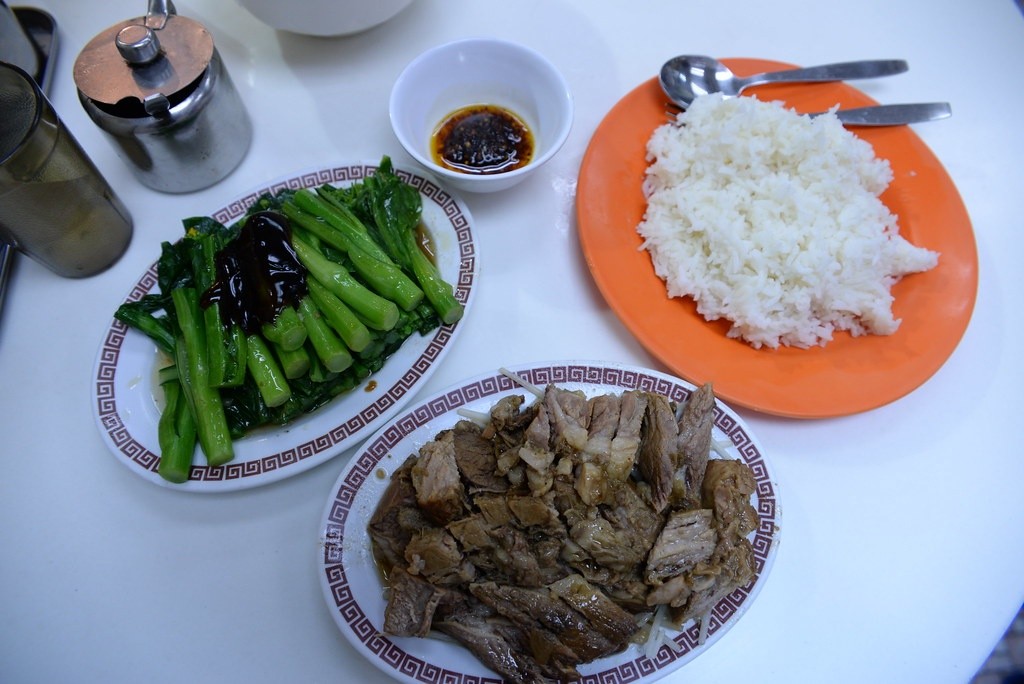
[660,54,908,110]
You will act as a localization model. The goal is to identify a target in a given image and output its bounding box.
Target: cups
[73,0,254,195]
[0,61,134,280]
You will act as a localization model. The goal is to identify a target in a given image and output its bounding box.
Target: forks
[665,103,953,128]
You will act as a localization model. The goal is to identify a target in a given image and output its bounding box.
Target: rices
[635,88,943,350]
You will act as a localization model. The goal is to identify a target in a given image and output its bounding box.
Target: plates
[318,359,783,684]
[240,1,416,37]
[576,58,978,421]
[92,160,481,493]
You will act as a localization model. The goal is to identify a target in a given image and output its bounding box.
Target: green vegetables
[114,151,465,482]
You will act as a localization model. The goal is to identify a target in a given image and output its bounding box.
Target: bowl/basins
[389,37,573,193]
